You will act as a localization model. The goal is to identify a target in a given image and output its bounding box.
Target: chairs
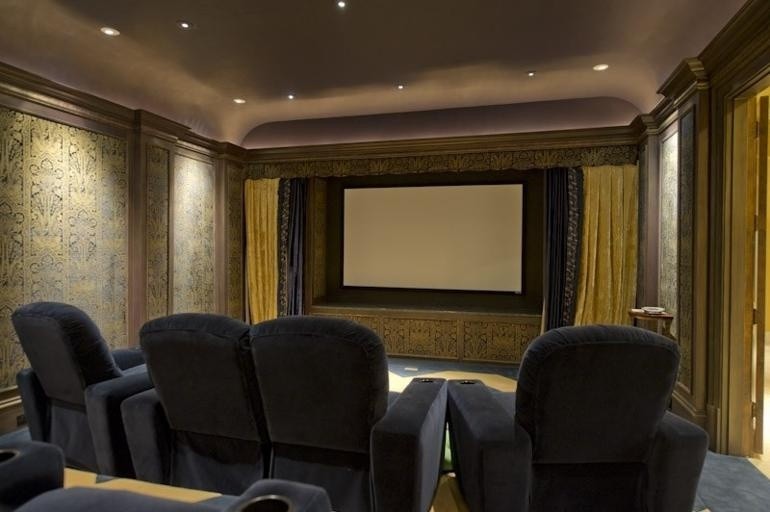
[117,310,447,512]
[448,317,709,510]
[0,434,335,512]
[9,299,155,480]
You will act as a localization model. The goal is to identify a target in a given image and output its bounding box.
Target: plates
[642,307,666,314]
